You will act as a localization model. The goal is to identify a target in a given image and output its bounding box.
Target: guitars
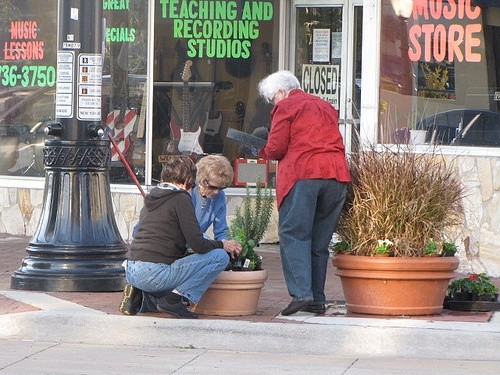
[248,41,273,133]
[226,37,255,78]
[105,88,137,162]
[165,59,203,163]
[126,81,148,186]
[170,36,201,91]
[234,102,245,131]
[199,57,224,154]
[154,49,170,138]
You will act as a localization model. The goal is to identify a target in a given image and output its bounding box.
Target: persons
[118,155,230,319]
[132,156,242,259]
[258,70,351,316]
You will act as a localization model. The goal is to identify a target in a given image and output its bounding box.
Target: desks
[154,82,214,129]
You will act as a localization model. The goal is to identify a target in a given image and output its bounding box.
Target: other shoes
[298,304,326,314]
[281,299,307,315]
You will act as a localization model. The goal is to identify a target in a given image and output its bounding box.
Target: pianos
[154,81,233,130]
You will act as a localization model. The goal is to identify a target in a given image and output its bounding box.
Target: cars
[414,108,500,147]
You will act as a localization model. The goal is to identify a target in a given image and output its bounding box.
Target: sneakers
[157,294,199,319]
[119,284,141,316]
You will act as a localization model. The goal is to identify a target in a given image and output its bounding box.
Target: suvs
[0,74,147,176]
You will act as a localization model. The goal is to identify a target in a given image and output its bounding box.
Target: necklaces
[199,197,209,209]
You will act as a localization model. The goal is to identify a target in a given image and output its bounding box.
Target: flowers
[450,271,497,298]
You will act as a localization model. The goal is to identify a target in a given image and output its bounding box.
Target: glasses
[206,181,228,190]
[191,182,196,190]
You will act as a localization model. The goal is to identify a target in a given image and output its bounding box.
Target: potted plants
[189,175,276,316]
[332,107,481,315]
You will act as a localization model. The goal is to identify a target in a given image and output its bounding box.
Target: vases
[454,292,497,302]
